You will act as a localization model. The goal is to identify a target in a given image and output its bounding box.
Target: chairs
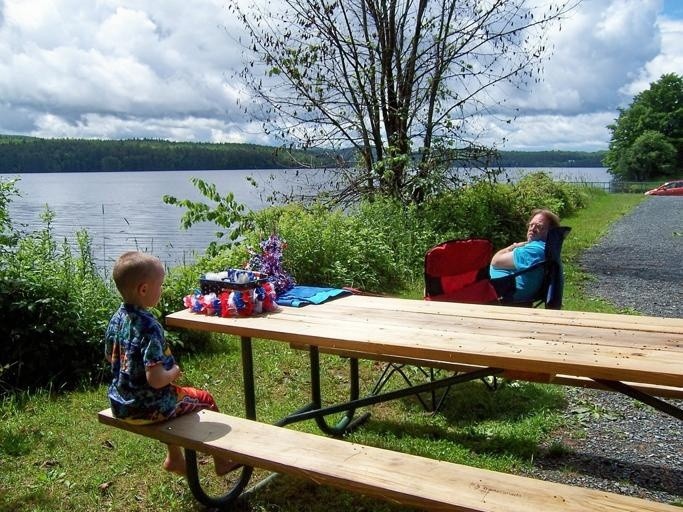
[372,238,499,412]
[491,227,571,309]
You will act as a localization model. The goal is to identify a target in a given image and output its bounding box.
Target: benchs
[98,406,683,512]
[290,342,683,434]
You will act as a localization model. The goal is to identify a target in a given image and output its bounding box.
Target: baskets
[199,269,268,296]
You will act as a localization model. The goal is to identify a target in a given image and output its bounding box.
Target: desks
[164,294,683,507]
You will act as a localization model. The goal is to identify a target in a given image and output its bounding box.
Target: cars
[645,179,682,197]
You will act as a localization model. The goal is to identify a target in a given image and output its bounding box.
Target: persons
[104,250,242,477]
[487,208,557,304]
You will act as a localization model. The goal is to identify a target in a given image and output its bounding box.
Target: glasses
[526,223,547,231]
